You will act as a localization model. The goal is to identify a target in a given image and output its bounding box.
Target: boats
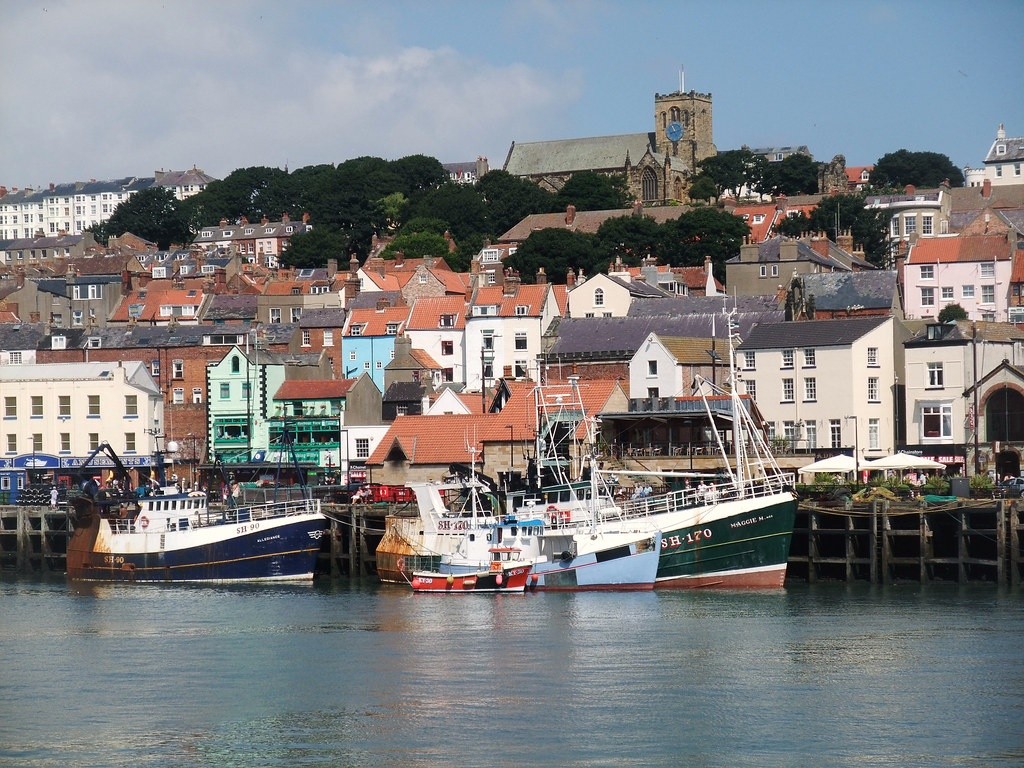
[60,396,330,589]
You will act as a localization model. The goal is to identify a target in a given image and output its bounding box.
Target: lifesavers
[139,516,149,528]
[559,511,570,520]
[546,505,559,518]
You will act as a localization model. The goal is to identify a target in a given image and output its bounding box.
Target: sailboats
[399,302,795,598]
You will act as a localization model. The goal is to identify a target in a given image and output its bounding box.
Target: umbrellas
[798,452,869,483]
[863,454,948,484]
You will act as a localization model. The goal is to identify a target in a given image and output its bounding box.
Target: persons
[686,479,716,501]
[632,483,653,499]
[119,505,129,530]
[222,482,242,508]
[917,470,929,485]
[49,486,59,509]
[351,487,370,504]
[756,468,765,483]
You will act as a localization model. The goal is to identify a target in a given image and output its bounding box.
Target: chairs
[625,441,769,456]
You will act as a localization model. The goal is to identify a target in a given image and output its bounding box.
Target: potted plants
[598,441,619,459]
[771,435,789,454]
